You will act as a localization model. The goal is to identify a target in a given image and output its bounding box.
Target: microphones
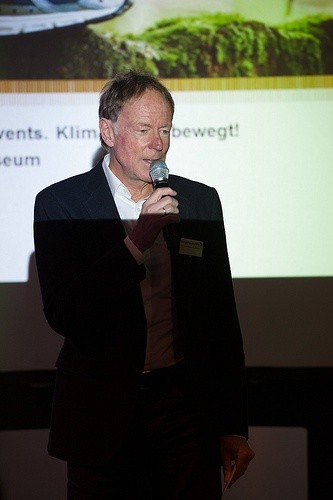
[149,160,176,249]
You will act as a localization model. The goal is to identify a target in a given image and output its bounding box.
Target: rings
[162,207,167,214]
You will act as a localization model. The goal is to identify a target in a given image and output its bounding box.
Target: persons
[34,72,255,500]
